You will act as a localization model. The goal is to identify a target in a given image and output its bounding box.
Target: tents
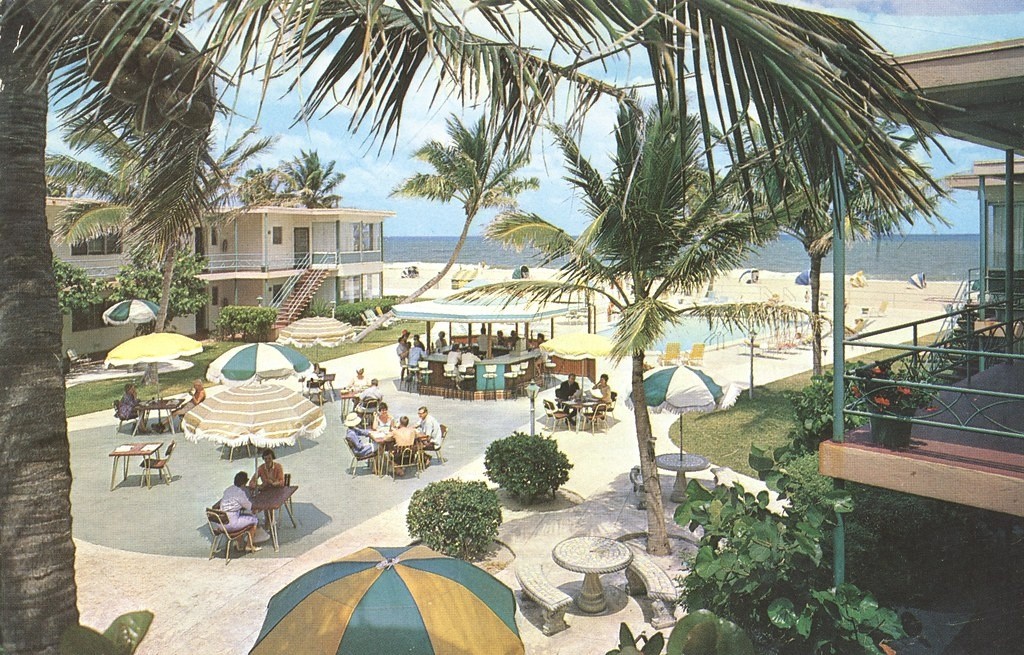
[511,265,531,280]
[794,270,811,285]
[401,266,419,279]
[738,269,759,284]
[906,272,927,290]
[848,270,868,288]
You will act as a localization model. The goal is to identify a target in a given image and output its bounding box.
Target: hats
[401,330,410,338]
[344,412,362,427]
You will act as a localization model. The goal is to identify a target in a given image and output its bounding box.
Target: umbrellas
[538,334,633,399]
[102,299,160,337]
[276,315,354,363]
[246,544,526,654]
[104,333,203,424]
[206,341,314,387]
[180,382,326,521]
[625,363,741,461]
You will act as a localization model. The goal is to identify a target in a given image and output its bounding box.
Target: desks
[368,431,428,477]
[552,535,634,614]
[108,442,164,492]
[656,453,709,503]
[562,399,602,433]
[298,374,336,404]
[211,484,298,552]
[340,389,362,422]
[131,398,185,437]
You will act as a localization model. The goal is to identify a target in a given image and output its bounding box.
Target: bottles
[152,398,155,405]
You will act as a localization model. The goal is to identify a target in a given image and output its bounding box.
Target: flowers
[850,360,939,414]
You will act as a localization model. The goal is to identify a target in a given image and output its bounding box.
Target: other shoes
[245,545,262,552]
[151,422,165,434]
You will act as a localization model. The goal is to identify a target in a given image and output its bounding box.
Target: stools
[402,359,557,402]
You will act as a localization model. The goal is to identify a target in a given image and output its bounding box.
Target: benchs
[625,556,679,630]
[515,564,571,638]
[710,465,740,490]
[630,465,648,510]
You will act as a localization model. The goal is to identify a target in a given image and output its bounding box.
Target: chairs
[657,342,707,367]
[66,348,91,373]
[544,390,617,433]
[734,300,890,360]
[359,306,407,330]
[112,367,448,565]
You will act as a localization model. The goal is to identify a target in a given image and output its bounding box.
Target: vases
[867,406,915,448]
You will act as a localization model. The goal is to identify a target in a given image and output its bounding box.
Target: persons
[119,383,149,432]
[343,366,442,477]
[445,343,482,392]
[249,448,285,532]
[397,329,426,384]
[435,331,446,349]
[534,334,548,378]
[217,472,262,551]
[479,327,519,360]
[557,372,612,426]
[302,363,328,403]
[151,379,206,434]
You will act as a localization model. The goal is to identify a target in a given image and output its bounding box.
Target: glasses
[418,412,425,415]
